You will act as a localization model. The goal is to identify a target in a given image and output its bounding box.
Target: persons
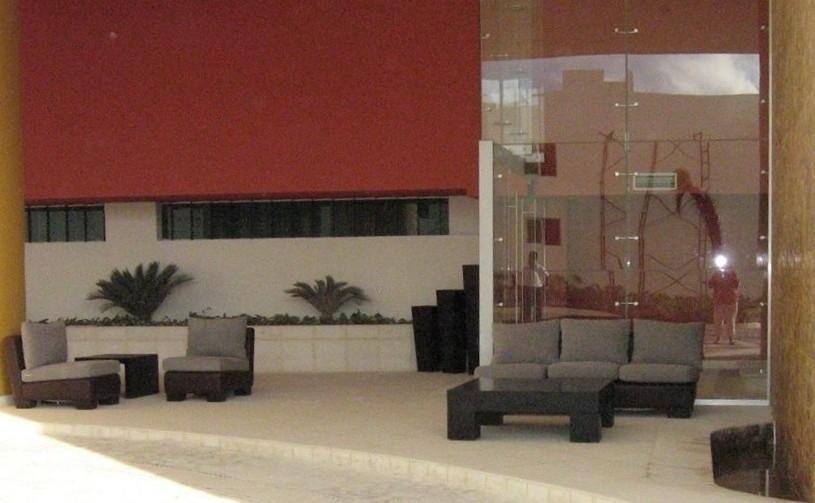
[515,250,551,323]
[705,264,742,345]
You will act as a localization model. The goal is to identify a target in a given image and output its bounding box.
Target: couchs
[474,316,705,418]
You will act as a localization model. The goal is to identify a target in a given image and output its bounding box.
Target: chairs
[165,315,254,401]
[5,320,121,406]
